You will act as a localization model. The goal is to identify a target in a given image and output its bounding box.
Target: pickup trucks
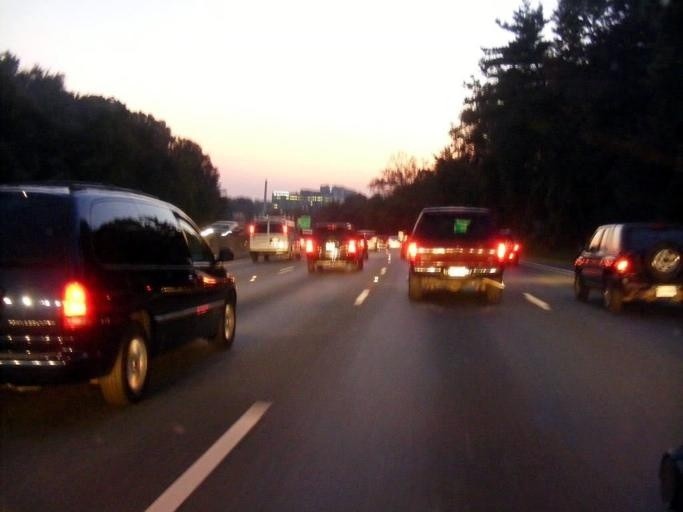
[298,219,370,274]
[401,203,519,306]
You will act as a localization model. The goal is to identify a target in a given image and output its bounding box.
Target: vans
[248,217,301,263]
[0,181,241,403]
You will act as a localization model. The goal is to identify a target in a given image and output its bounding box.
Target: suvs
[570,221,682,316]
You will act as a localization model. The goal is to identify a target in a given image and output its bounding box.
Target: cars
[198,220,246,262]
[355,226,408,252]
